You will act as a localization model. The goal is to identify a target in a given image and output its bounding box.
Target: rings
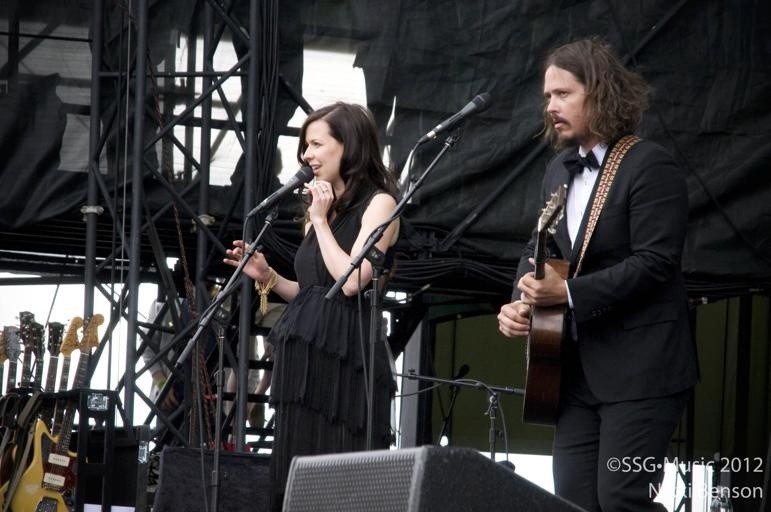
[323,186,329,194]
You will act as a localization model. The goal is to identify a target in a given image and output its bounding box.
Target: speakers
[152,444,273,512]
[281,444,589,511]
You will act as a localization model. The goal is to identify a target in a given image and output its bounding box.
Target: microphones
[245,165,314,217]
[449,362,471,392]
[415,90,493,143]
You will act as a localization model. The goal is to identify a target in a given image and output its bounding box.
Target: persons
[497,38,704,510]
[142,252,230,448]
[224,102,404,511]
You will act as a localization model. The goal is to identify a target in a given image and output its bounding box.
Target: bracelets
[157,379,168,391]
[256,268,277,294]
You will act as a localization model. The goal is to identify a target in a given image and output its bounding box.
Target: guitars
[0,311,105,512]
[522,184,568,427]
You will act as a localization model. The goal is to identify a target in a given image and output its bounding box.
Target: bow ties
[562,150,599,175]
